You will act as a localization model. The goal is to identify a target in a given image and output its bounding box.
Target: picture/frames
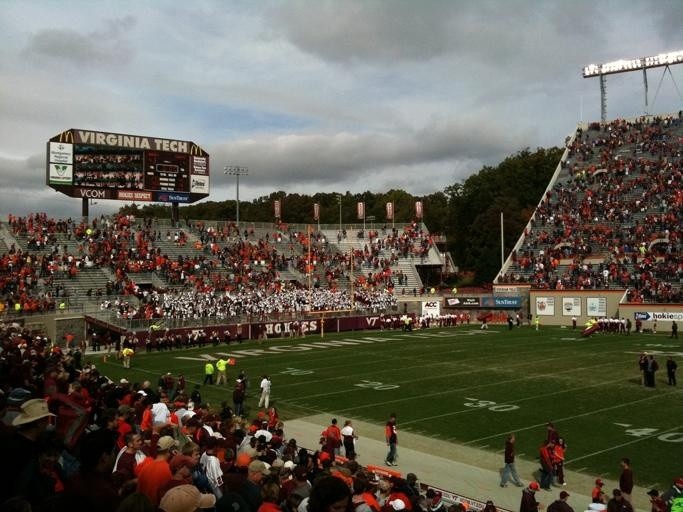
[535,296,555,316]
[562,297,582,317]
[586,297,607,317]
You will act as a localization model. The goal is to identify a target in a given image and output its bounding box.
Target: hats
[7,389,31,405]
[248,458,297,476]
[157,484,217,512]
[120,379,128,383]
[560,491,569,498]
[118,405,135,414]
[647,489,658,496]
[157,435,181,452]
[596,479,602,485]
[529,482,540,491]
[675,478,683,484]
[138,391,147,395]
[12,398,57,426]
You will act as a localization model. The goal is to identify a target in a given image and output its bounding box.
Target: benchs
[502,122,683,305]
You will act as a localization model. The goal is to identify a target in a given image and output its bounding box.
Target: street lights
[221,164,250,229]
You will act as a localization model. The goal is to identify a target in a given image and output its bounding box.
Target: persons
[498,110,682,338]
[73,153,144,189]
[639,354,678,387]
[343,413,682,512]
[1,209,471,370]
[0,358,354,511]
[481,317,489,330]
